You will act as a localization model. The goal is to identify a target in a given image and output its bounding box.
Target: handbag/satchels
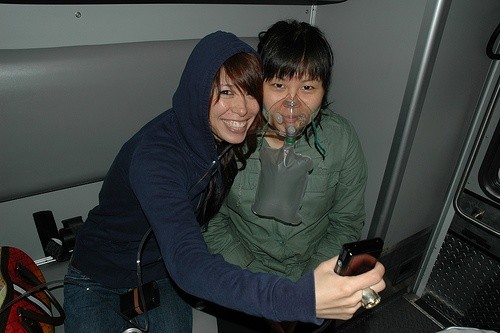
[0,245,65,333]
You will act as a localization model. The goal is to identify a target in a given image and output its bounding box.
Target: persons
[63,30,386,333]
[200,20,368,333]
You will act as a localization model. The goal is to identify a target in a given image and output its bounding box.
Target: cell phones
[333,237,384,277]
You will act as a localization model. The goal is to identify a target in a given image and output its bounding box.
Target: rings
[361,288,381,310]
